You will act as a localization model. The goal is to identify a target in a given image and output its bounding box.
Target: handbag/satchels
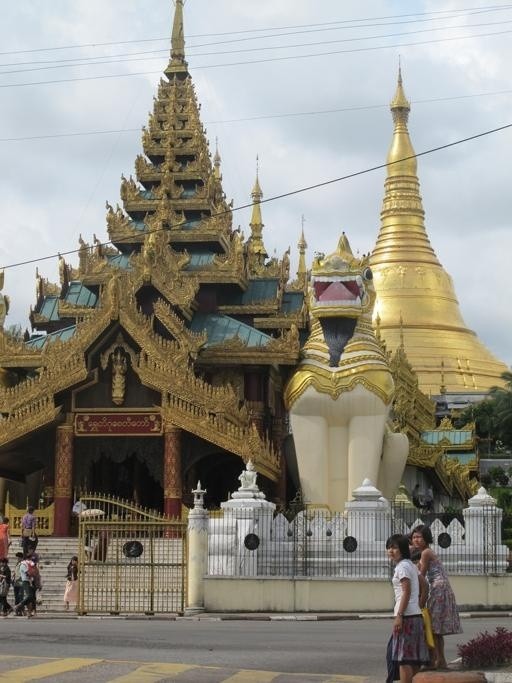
[0,578,10,595]
[421,607,434,647]
[22,527,34,537]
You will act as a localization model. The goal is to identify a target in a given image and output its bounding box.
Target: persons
[383,533,435,683]
[237,458,258,488]
[425,483,436,512]
[0,505,114,616]
[411,524,465,671]
[387,549,436,671]
[412,482,421,507]
[402,532,417,555]
